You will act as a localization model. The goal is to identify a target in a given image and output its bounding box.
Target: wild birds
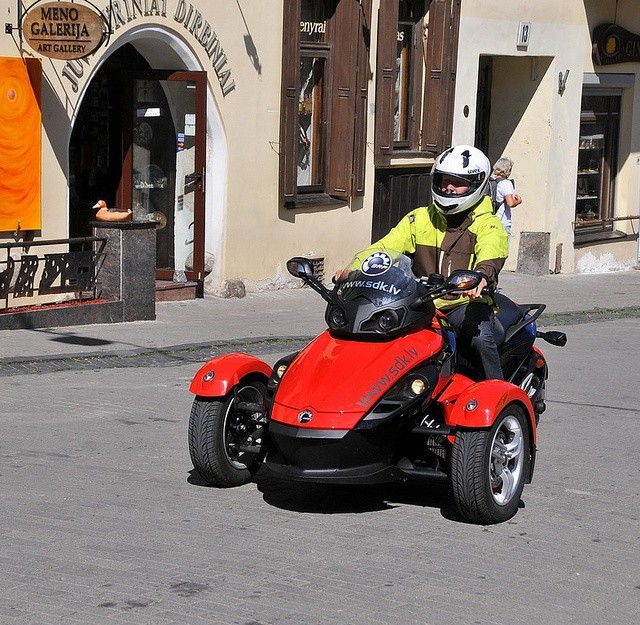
[91,199,133,222]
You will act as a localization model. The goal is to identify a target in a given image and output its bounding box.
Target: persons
[484,157,522,247]
[335,145,509,380]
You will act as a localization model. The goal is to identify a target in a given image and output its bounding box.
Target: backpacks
[489,177,515,214]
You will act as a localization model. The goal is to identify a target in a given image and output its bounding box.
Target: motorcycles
[187,254,568,527]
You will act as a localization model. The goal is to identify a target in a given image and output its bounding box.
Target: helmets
[430,144,492,216]
